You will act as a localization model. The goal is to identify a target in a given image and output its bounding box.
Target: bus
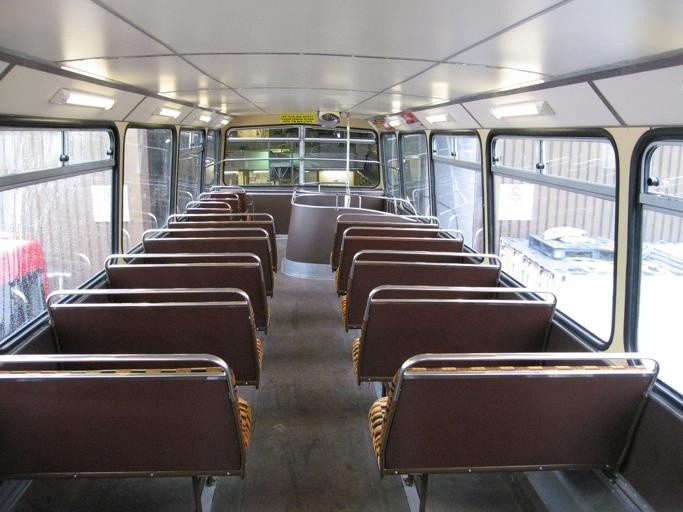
[0,237,49,343]
